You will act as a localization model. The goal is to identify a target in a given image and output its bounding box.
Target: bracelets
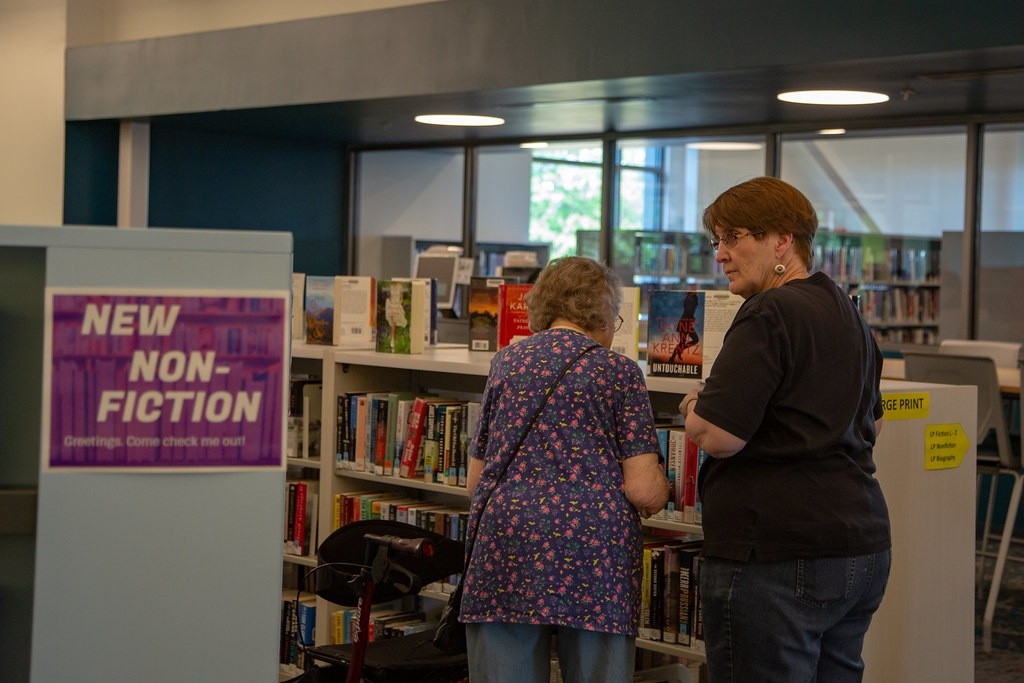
[684,397,698,419]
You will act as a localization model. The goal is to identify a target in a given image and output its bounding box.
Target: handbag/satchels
[432,584,465,650]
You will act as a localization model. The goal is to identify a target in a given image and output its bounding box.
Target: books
[280,231,941,683]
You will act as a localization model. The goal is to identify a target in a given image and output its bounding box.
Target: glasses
[710,229,793,252]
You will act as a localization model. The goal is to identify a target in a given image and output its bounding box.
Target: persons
[679,176,893,683]
[457,255,671,683]
[669,292,699,364]
[385,282,407,348]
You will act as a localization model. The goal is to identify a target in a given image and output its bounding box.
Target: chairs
[901,349,1024,655]
[938,337,1024,587]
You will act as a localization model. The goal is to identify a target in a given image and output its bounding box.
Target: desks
[879,357,1024,393]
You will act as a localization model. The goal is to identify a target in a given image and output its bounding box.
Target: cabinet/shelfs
[634,230,944,352]
[278,336,331,683]
[0,222,294,683]
[320,342,980,683]
